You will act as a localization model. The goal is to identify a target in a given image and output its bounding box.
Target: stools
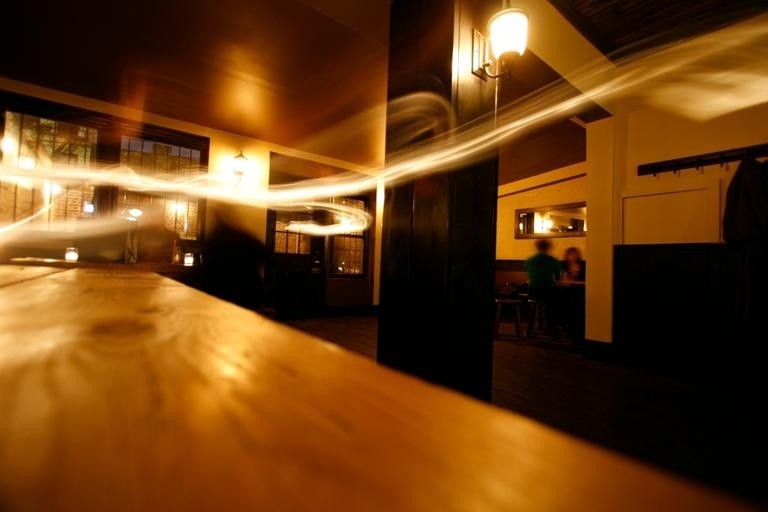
[491,295,558,343]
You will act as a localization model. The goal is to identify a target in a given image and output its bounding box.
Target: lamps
[471,7,528,83]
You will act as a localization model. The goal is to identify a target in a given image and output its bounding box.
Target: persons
[198,206,268,310]
[523,240,564,336]
[562,248,585,280]
